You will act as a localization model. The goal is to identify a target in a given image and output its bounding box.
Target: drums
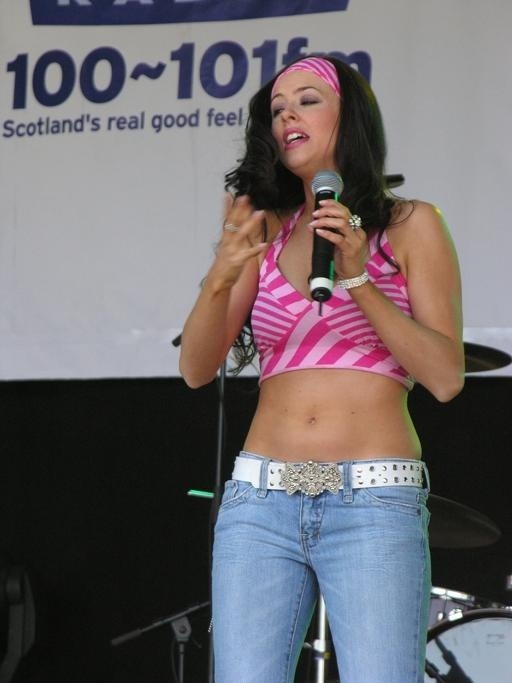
[424,604,511,682]
[427,585,500,630]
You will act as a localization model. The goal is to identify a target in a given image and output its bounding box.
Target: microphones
[307,167,346,301]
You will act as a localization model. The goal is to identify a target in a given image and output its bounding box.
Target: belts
[230,455,423,497]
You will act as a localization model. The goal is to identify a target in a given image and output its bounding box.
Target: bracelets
[337,269,369,289]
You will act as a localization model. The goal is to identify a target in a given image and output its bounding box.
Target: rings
[348,214,362,231]
[224,224,238,233]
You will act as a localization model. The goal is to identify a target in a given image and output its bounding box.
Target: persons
[179,57,464,683]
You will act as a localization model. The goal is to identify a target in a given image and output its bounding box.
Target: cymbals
[427,494,502,547]
[463,343,511,372]
